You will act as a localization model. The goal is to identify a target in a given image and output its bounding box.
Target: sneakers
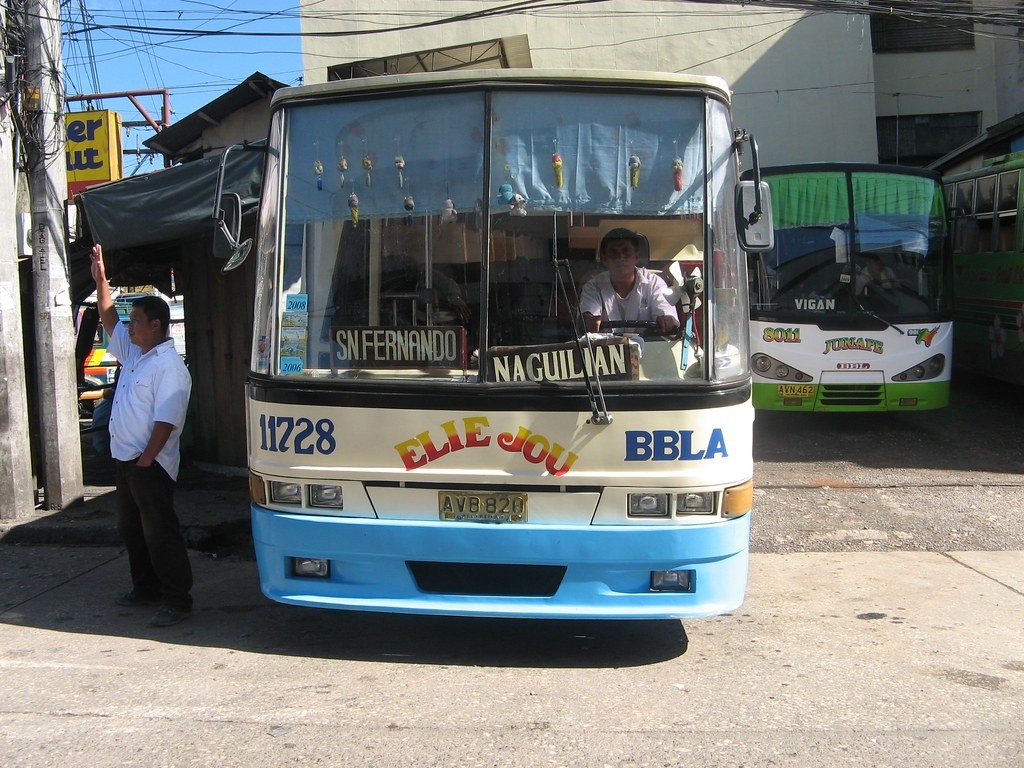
[116,588,158,605]
[149,605,192,626]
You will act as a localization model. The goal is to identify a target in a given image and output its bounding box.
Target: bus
[738,162,982,417]
[210,66,776,620]
[935,150,1024,397]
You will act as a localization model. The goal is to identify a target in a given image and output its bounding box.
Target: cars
[78,321,124,431]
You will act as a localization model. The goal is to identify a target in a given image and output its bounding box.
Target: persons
[576,228,680,334]
[90,244,193,628]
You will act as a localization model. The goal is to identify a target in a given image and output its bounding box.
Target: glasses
[606,247,635,259]
